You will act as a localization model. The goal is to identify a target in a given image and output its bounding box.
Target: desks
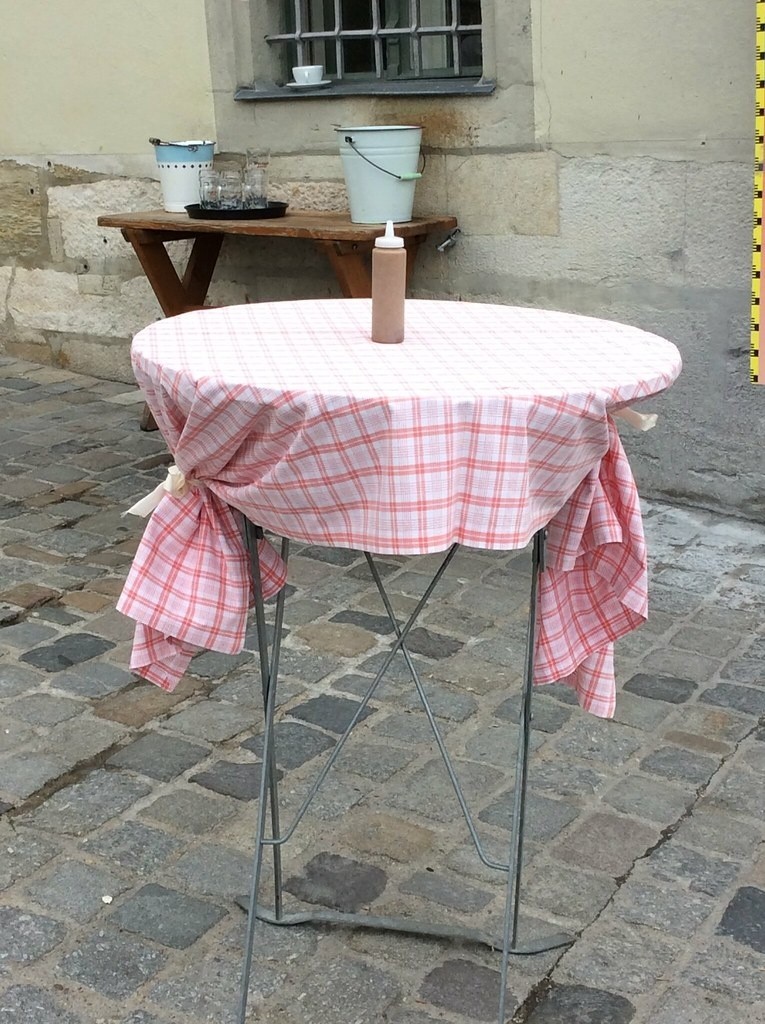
[116,298,684,1024]
[96,207,456,432]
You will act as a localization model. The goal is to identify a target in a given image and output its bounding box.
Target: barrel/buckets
[333,125,424,224]
[149,137,217,212]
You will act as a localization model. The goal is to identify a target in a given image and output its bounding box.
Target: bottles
[372,219,407,344]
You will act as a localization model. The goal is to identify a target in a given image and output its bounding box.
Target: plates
[286,79,332,88]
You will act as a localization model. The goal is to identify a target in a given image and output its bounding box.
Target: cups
[197,145,272,210]
[291,65,324,83]
